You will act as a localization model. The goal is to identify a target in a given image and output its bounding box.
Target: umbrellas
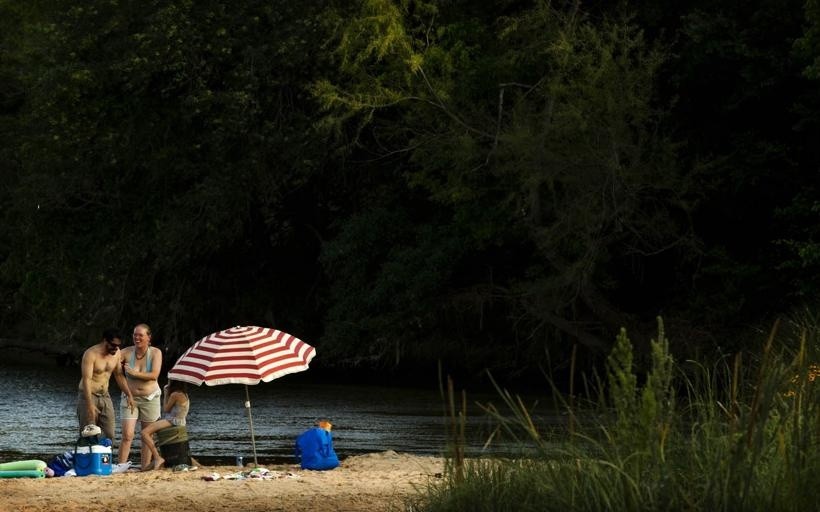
[168,326,317,469]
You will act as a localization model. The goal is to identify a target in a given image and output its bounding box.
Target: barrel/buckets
[153,425,192,468]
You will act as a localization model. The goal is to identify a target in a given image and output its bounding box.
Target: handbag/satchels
[294,428,341,471]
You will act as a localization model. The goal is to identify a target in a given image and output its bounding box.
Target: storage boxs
[76,445,112,477]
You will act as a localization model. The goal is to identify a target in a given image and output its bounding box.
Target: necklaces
[135,348,148,360]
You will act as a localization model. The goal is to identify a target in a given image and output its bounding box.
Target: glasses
[108,340,122,348]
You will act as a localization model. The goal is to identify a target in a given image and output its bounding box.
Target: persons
[141,381,200,469]
[76,327,135,448]
[118,324,162,469]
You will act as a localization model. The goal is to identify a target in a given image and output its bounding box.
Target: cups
[236,456,243,467]
